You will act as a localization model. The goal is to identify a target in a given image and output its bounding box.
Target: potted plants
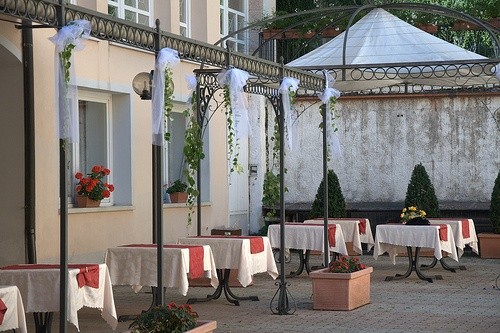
[478,170,500,259]
[396,163,446,256]
[166,180,188,203]
[310,169,356,254]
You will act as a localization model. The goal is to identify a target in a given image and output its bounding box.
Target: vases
[78,195,100,207]
[309,267,373,311]
[122,320,217,333]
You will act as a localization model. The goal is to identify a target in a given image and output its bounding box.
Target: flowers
[130,303,200,333]
[75,165,114,202]
[400,206,430,226]
[329,255,366,273]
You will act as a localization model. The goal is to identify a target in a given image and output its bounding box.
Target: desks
[0,284,27,333]
[267,222,348,278]
[421,218,478,273]
[304,217,375,270]
[103,244,220,323]
[373,223,458,283]
[176,236,280,306]
[0,264,118,333]
